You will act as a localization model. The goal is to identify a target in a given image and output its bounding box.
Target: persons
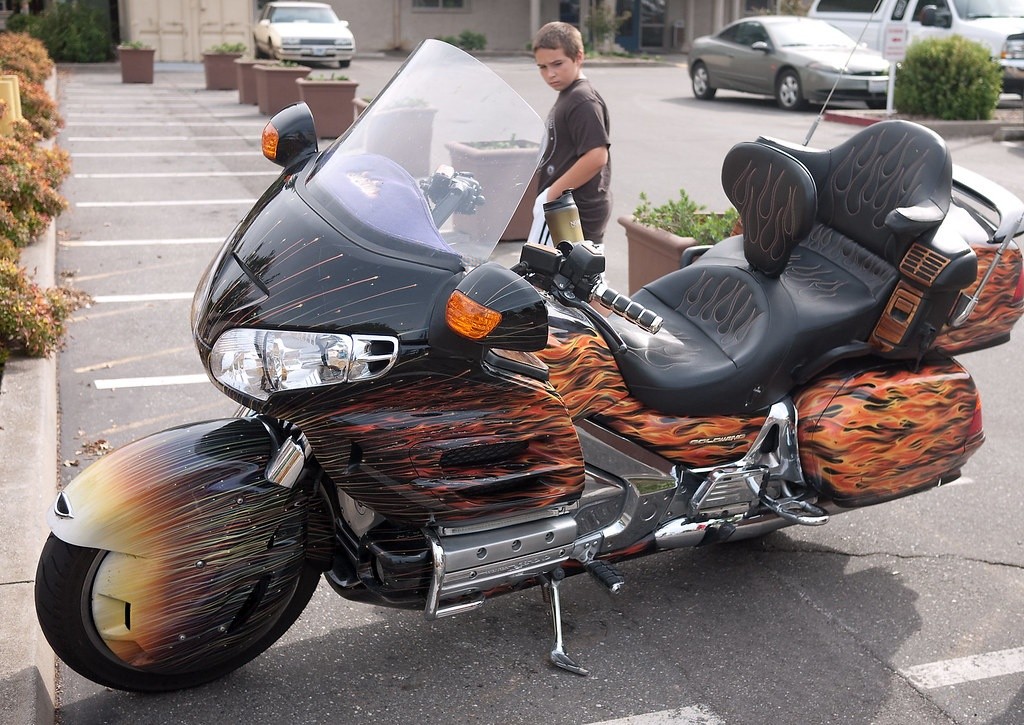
[526,21,613,249]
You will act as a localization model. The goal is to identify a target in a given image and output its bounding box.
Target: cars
[253,2,356,68]
[687,16,891,113]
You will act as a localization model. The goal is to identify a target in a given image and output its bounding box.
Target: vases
[233,58,282,104]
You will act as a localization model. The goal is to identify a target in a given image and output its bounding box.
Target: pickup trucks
[809,0,1024,102]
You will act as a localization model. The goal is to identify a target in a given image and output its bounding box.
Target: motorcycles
[35,39,1024,699]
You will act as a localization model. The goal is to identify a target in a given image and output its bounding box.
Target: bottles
[542,189,585,248]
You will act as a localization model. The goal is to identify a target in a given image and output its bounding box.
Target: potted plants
[117,40,156,83]
[617,188,736,299]
[201,42,247,91]
[253,59,313,116]
[445,132,542,242]
[296,73,360,138]
[351,95,439,177]
